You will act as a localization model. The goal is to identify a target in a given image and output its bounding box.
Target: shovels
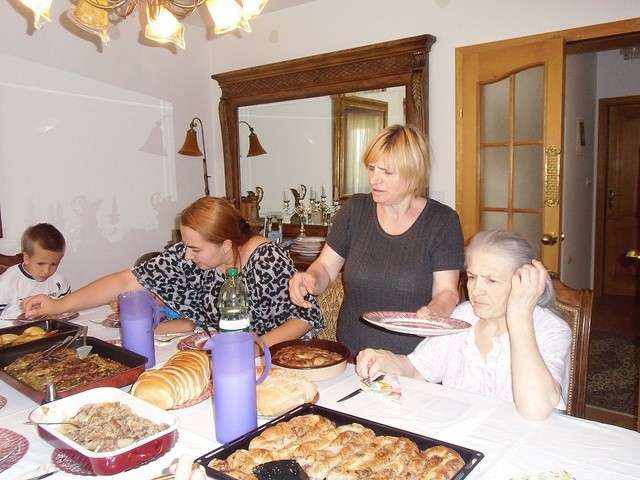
[77,326,93,359]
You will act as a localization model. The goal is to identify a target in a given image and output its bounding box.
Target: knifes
[336,373,386,403]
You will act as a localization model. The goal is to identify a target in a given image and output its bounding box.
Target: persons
[0,223,78,318]
[22,196,328,359]
[288,123,465,364]
[355,228,573,421]
[109,252,197,336]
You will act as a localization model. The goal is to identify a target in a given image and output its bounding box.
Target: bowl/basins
[269,339,351,381]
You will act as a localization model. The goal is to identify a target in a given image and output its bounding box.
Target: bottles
[216,267,250,334]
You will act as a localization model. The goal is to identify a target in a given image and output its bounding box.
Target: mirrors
[211,34,437,237]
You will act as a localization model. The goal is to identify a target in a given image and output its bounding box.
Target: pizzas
[9,343,125,393]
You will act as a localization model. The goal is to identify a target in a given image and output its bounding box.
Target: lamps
[177,117,216,197]
[238,119,268,157]
[12,0,269,48]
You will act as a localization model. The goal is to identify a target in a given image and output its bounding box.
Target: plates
[254,391,320,421]
[291,236,326,257]
[27,386,181,476]
[16,311,82,323]
[1,310,83,361]
[2,428,21,461]
[360,310,473,337]
[148,356,213,413]
[0,334,149,403]
[108,308,168,323]
[182,331,220,353]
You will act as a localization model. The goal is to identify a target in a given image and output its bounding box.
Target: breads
[210,448,268,480]
[273,345,343,367]
[290,421,376,480]
[329,435,419,479]
[396,444,465,480]
[23,325,52,336]
[1,332,30,346]
[256,365,318,420]
[132,350,213,410]
[249,411,335,461]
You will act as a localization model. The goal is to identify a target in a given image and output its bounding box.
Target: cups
[203,331,272,446]
[117,290,161,370]
